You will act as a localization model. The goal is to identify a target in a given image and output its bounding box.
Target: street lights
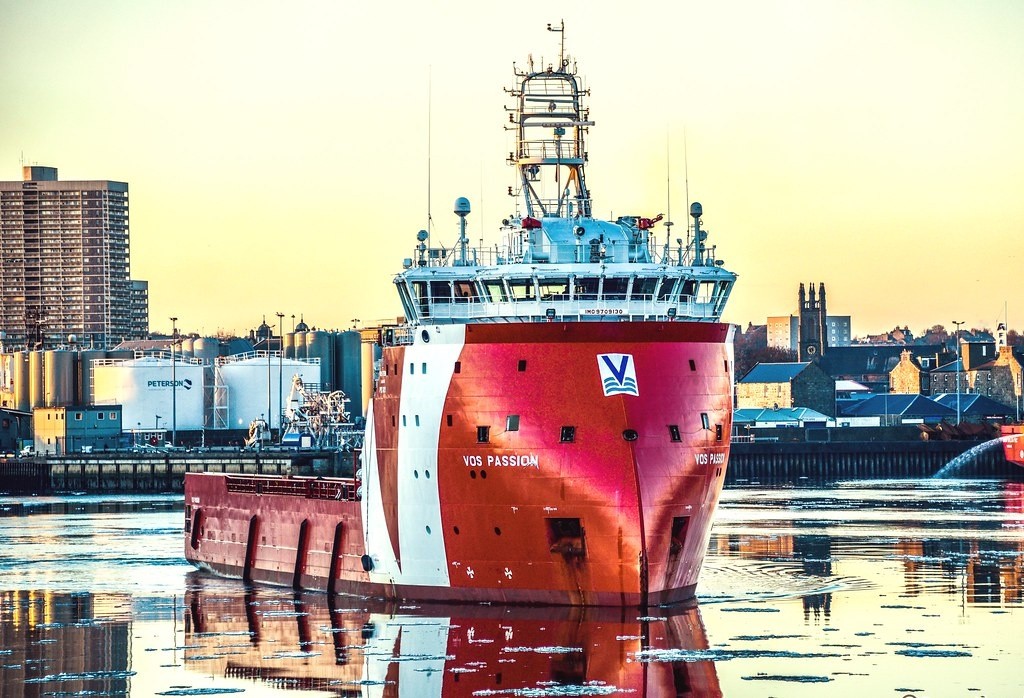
[953,320,965,426]
[264,324,271,433]
[169,317,178,447]
[275,312,285,446]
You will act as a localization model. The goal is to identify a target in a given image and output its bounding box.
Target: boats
[1001,425,1024,467]
[182,18,738,608]
[183,570,723,698]
[1001,484,1024,528]
